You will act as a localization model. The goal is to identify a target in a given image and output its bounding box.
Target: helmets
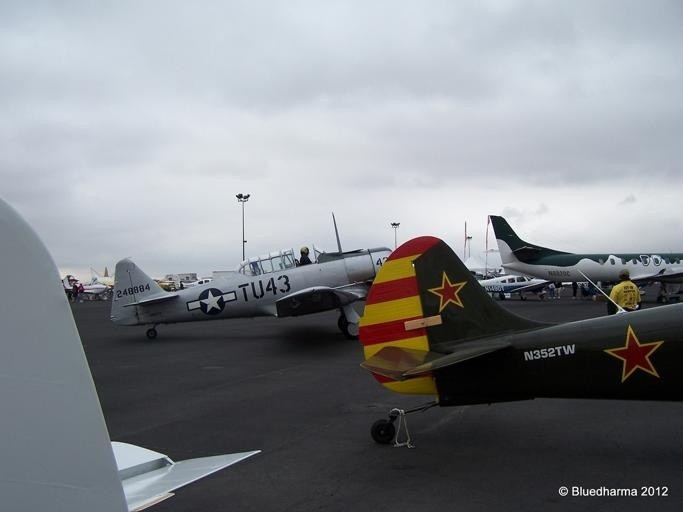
[300,247,309,255]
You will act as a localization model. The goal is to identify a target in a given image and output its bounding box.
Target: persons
[606,269,642,316]
[299,245,311,267]
[71,282,78,303]
[76,283,84,303]
[180,280,184,289]
[547,282,595,302]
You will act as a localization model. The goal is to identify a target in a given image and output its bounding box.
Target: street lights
[236,193,251,277]
